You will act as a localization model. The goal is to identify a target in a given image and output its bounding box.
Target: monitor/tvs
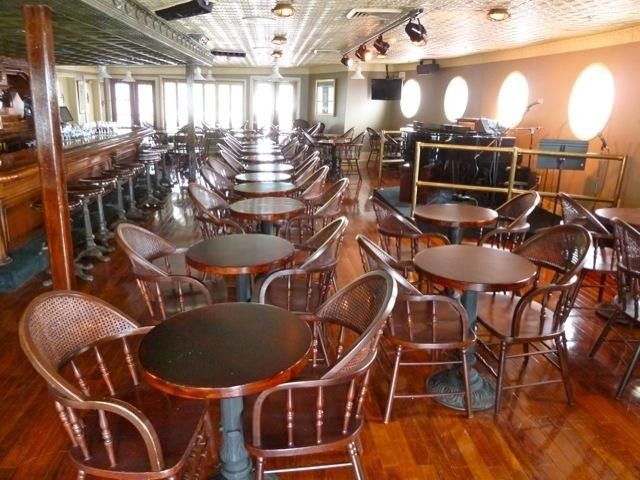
[372,79,401,99]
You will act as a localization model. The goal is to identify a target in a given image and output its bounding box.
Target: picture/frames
[314,78,338,117]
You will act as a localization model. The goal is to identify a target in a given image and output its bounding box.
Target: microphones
[527,99,544,108]
[598,132,608,149]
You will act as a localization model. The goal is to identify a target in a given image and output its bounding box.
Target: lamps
[121,67,136,83]
[272,3,296,18]
[205,69,216,82]
[486,8,512,23]
[339,7,427,68]
[193,66,206,81]
[271,51,282,58]
[96,66,114,80]
[267,63,284,82]
[349,66,366,80]
[271,36,289,46]
